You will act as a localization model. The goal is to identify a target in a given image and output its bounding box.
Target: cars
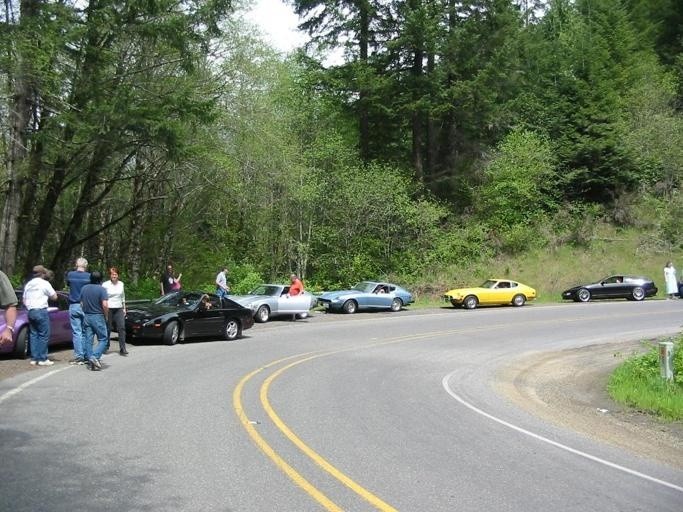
[673,280,682,298]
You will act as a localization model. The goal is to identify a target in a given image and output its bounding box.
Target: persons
[0,268,19,347]
[172,271,183,291]
[201,294,212,310]
[79,270,108,371]
[213,265,230,297]
[64,257,91,366]
[615,278,622,284]
[375,286,385,295]
[675,276,683,299]
[101,267,129,355]
[21,264,58,367]
[160,262,173,297]
[288,273,303,320]
[663,261,679,300]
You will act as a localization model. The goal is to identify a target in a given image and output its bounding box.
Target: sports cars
[316,280,414,315]
[439,278,537,309]
[223,281,318,323]
[0,287,73,360]
[111,287,255,346]
[560,273,659,303]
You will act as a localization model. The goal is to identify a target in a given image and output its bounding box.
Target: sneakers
[69,355,102,371]
[119,349,129,355]
[30,359,54,366]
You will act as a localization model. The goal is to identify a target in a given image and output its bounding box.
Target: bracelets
[6,326,15,334]
